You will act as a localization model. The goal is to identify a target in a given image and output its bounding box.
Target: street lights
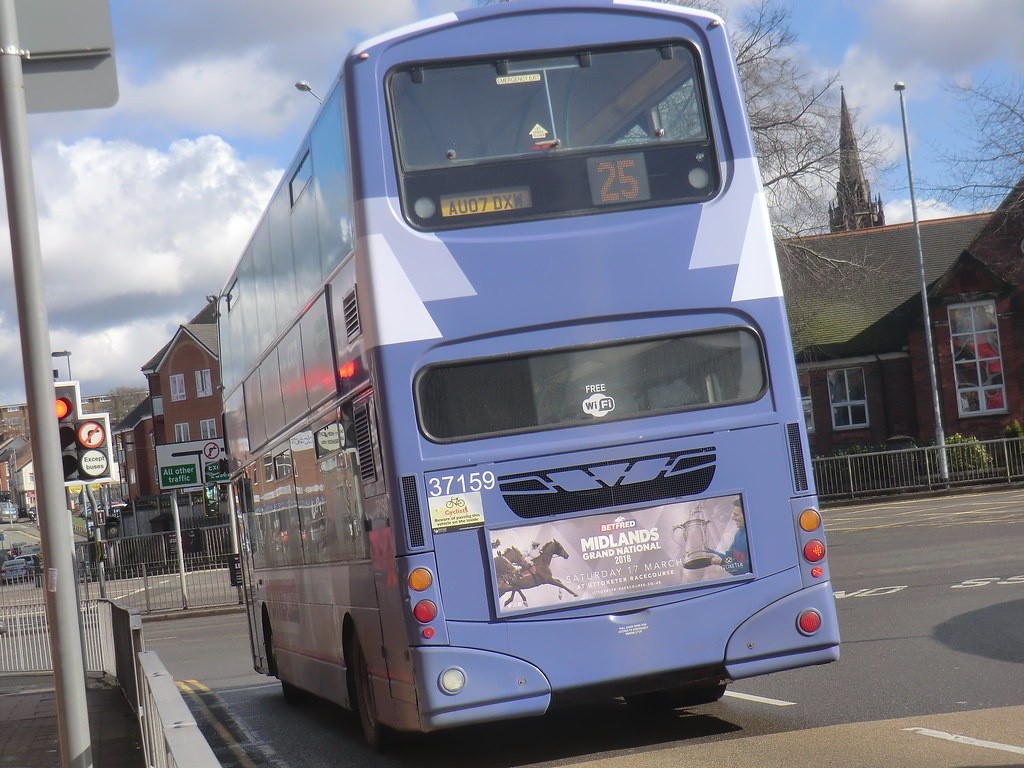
[52,350,90,543]
[894,83,951,492]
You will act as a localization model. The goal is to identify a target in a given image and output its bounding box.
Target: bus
[214,0,841,757]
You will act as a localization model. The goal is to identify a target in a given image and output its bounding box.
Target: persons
[704,497,751,576]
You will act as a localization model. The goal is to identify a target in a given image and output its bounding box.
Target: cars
[1,554,42,583]
[0,501,18,523]
[28,507,37,521]
[11,542,27,558]
[79,502,128,521]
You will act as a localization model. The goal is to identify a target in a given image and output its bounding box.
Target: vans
[305,517,327,547]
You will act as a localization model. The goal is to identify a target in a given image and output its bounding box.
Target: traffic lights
[54,380,113,484]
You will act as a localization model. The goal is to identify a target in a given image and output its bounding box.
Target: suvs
[0,491,11,501]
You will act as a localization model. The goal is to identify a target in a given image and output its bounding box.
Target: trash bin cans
[884,435,915,486]
[105,516,119,538]
[149,514,172,532]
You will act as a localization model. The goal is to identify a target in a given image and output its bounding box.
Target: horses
[490,537,579,607]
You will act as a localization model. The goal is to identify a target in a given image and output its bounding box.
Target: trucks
[21,544,40,556]
